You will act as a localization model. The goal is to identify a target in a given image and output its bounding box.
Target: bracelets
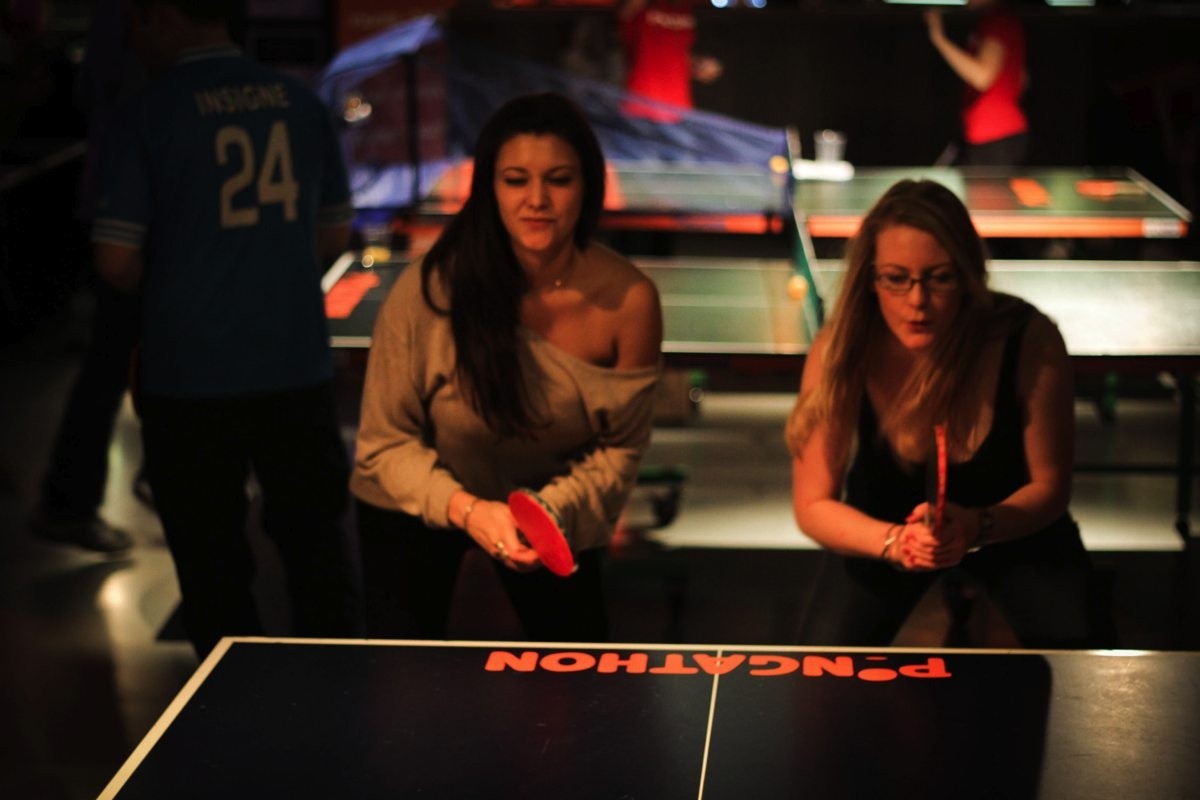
[977,506,992,544]
[463,497,480,531]
[879,525,897,560]
[896,526,904,560]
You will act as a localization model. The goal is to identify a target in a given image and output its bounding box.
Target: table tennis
[788,274,809,300]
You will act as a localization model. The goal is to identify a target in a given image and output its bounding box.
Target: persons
[348,93,665,641]
[921,0,1029,168]
[93,0,357,660]
[26,0,143,553]
[785,179,1110,650]
[619,0,720,142]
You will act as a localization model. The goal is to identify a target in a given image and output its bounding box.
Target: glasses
[876,271,963,296]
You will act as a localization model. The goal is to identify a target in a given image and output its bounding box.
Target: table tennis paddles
[508,488,577,576]
[926,423,946,537]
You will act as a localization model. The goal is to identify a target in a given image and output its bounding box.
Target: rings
[504,552,509,557]
[497,542,503,550]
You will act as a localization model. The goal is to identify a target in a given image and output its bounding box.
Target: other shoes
[25,499,133,555]
